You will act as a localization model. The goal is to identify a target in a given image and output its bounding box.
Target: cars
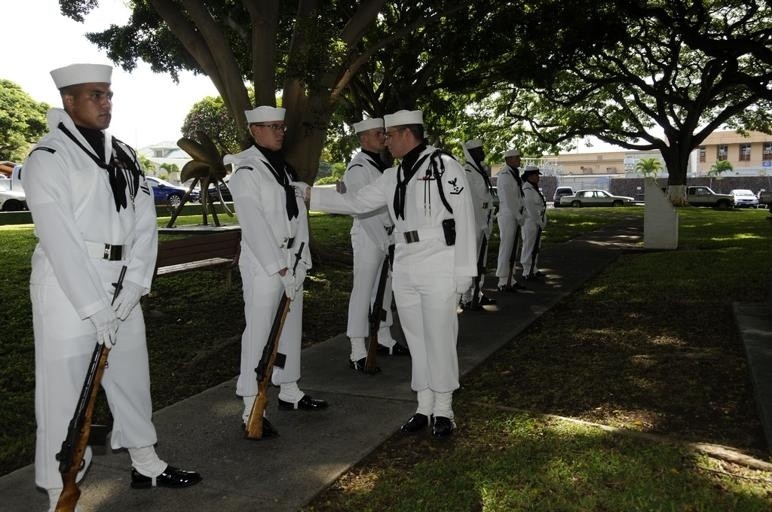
[729,189,758,209]
[0,162,30,213]
[554,186,635,207]
[143,175,232,205]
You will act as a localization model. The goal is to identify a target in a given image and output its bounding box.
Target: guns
[246,241,304,440]
[506,206,524,291]
[529,211,545,276]
[54,267,127,512]
[363,257,388,372]
[469,210,492,308]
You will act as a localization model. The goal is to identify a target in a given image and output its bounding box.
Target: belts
[84,240,127,261]
[279,237,295,249]
[481,202,490,209]
[384,224,395,235]
[394,227,445,243]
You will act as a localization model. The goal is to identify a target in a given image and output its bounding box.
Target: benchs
[153,229,241,293]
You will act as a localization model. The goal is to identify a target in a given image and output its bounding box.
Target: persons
[521,164,549,280]
[460,140,497,309]
[343,117,410,375]
[23,62,202,512]
[289,109,479,440]
[495,149,526,293]
[222,105,328,440]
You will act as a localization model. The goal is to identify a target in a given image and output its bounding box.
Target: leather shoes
[241,416,278,437]
[129,465,203,489]
[464,271,546,307]
[278,394,328,411]
[401,413,434,433]
[432,416,457,437]
[377,341,410,356]
[349,353,381,374]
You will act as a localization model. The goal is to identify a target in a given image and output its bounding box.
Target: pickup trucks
[758,191,772,210]
[662,186,734,211]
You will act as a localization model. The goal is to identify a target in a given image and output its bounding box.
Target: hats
[353,118,384,135]
[383,110,424,129]
[504,150,521,157]
[465,139,483,150]
[524,165,543,175]
[50,63,113,89]
[244,105,286,123]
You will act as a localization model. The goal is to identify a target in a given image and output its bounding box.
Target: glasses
[255,124,287,132]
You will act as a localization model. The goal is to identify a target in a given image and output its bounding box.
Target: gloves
[88,305,120,350]
[294,262,307,291]
[483,214,547,241]
[112,281,146,322]
[289,181,311,199]
[280,269,296,300]
[456,276,472,294]
